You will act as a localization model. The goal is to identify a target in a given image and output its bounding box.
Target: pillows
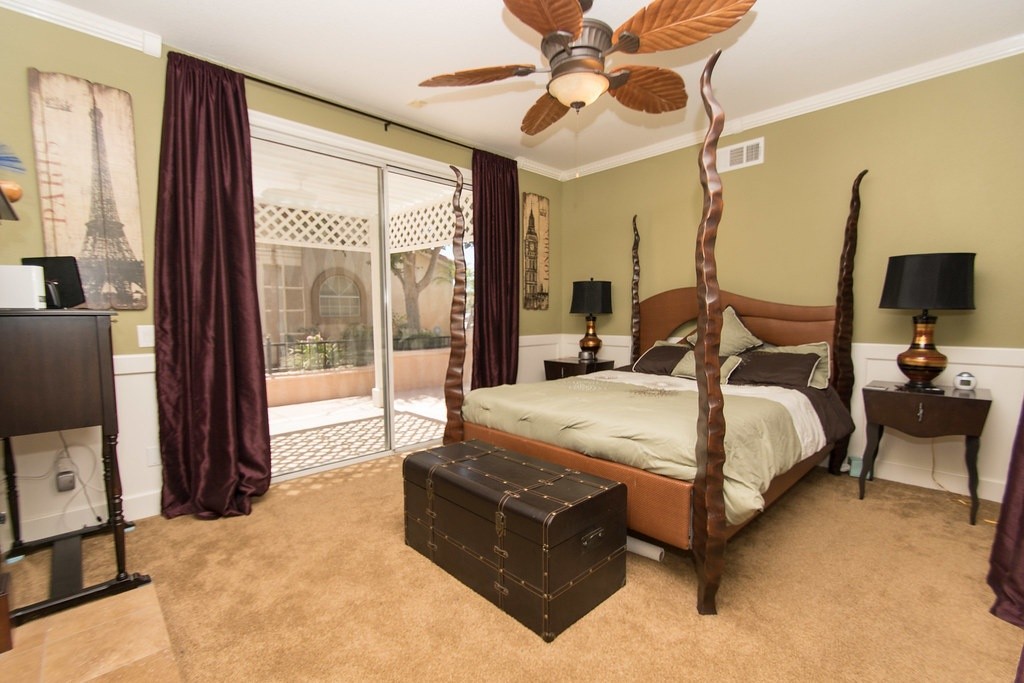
[686,304,763,359]
[632,340,742,385]
[728,350,822,389]
[752,341,830,389]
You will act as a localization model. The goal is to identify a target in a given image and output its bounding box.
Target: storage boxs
[402,439,628,644]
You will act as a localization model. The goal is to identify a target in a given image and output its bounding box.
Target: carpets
[270,411,446,483]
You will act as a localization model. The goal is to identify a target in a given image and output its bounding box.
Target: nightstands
[859,381,993,525]
[544,357,615,381]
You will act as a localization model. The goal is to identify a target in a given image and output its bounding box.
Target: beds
[461,287,860,616]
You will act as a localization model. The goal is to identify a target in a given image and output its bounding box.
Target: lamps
[569,278,612,356]
[546,57,610,113]
[879,253,976,388]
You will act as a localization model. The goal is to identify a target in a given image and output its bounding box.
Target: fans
[418,0,757,135]
